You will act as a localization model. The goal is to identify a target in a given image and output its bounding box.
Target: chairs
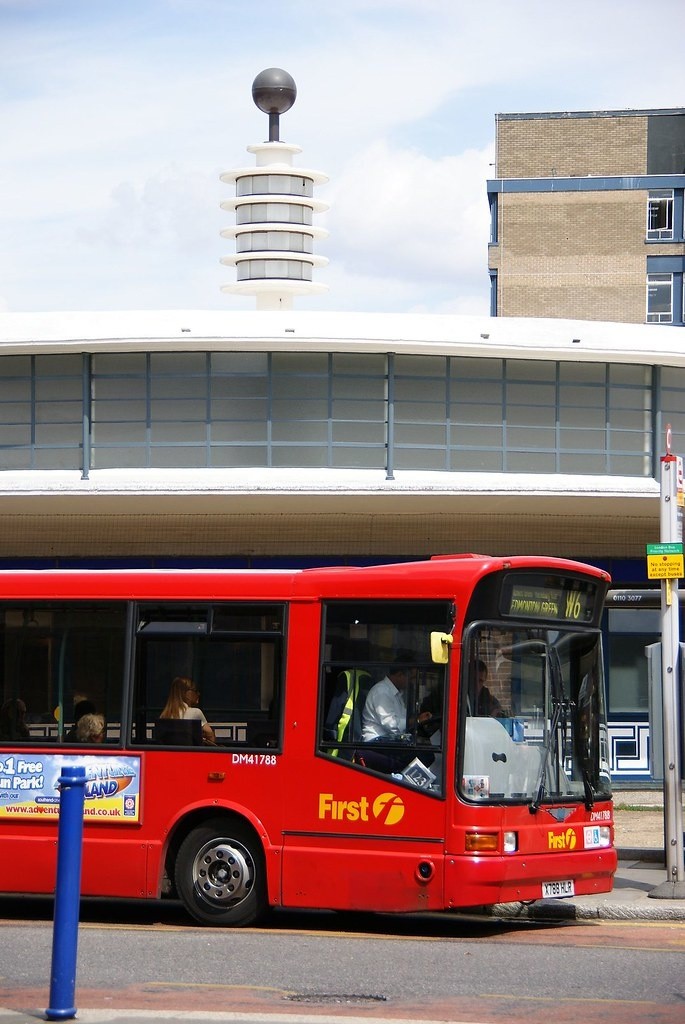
[325,669,373,763]
[153,716,202,746]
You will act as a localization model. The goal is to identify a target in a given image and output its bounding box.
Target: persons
[65,700,106,744]
[159,677,218,746]
[467,659,508,718]
[3,699,30,738]
[362,656,433,774]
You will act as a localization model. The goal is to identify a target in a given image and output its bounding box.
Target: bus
[0,553,610,926]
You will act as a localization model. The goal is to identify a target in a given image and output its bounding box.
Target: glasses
[187,688,201,692]
[479,679,486,684]
[404,670,417,680]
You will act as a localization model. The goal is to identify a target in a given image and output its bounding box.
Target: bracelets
[417,717,421,723]
